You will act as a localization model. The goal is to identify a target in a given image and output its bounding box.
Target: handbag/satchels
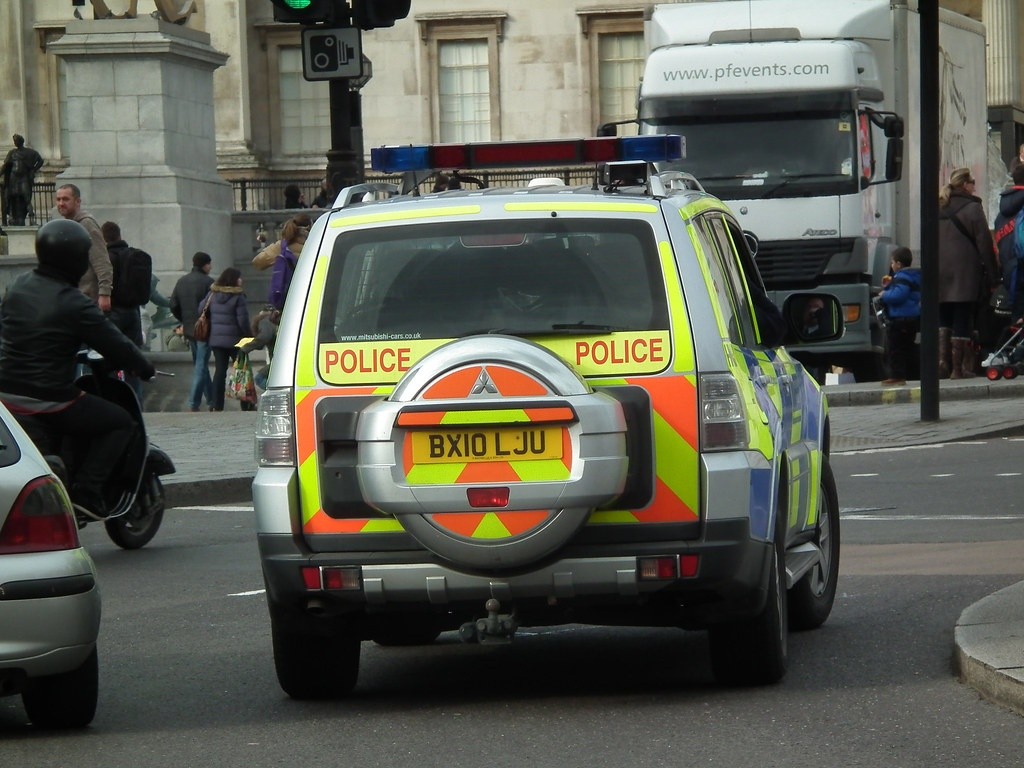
[989,281,1012,316]
[268,236,298,312]
[194,290,215,342]
[227,350,257,404]
[873,277,914,335]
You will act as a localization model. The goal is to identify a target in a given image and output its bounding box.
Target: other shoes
[881,375,906,388]
[75,487,110,518]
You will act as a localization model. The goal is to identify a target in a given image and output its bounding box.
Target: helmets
[36,219,93,285]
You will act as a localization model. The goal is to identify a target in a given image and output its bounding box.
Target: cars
[0,402,102,731]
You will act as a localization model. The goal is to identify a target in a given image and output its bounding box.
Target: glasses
[967,180,975,185]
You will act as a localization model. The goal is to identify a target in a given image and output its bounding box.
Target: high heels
[207,403,218,411]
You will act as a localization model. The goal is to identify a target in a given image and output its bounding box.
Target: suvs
[252,134,842,700]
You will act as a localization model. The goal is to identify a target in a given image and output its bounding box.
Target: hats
[1011,165,1024,185]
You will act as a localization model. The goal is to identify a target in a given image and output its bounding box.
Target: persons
[309,178,327,208]
[240,304,281,391]
[197,267,257,411]
[447,178,461,190]
[0,220,156,519]
[1009,144,1024,173]
[284,184,308,209]
[0,133,44,226]
[101,221,144,414]
[252,213,313,270]
[874,246,921,380]
[797,296,833,338]
[994,172,1024,317]
[939,166,1000,379]
[432,174,449,192]
[169,252,215,412]
[57,183,113,379]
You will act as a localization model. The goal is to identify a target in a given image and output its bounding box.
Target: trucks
[600,0,990,382]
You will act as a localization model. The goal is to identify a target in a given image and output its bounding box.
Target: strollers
[986,324,1024,381]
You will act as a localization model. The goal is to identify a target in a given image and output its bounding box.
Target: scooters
[36,351,176,550]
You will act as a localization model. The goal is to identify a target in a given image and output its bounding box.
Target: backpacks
[110,245,152,309]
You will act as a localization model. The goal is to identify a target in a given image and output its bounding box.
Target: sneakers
[998,355,1010,365]
[981,353,1004,366]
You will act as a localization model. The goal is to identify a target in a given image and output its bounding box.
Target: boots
[947,337,977,380]
[937,327,951,380]
